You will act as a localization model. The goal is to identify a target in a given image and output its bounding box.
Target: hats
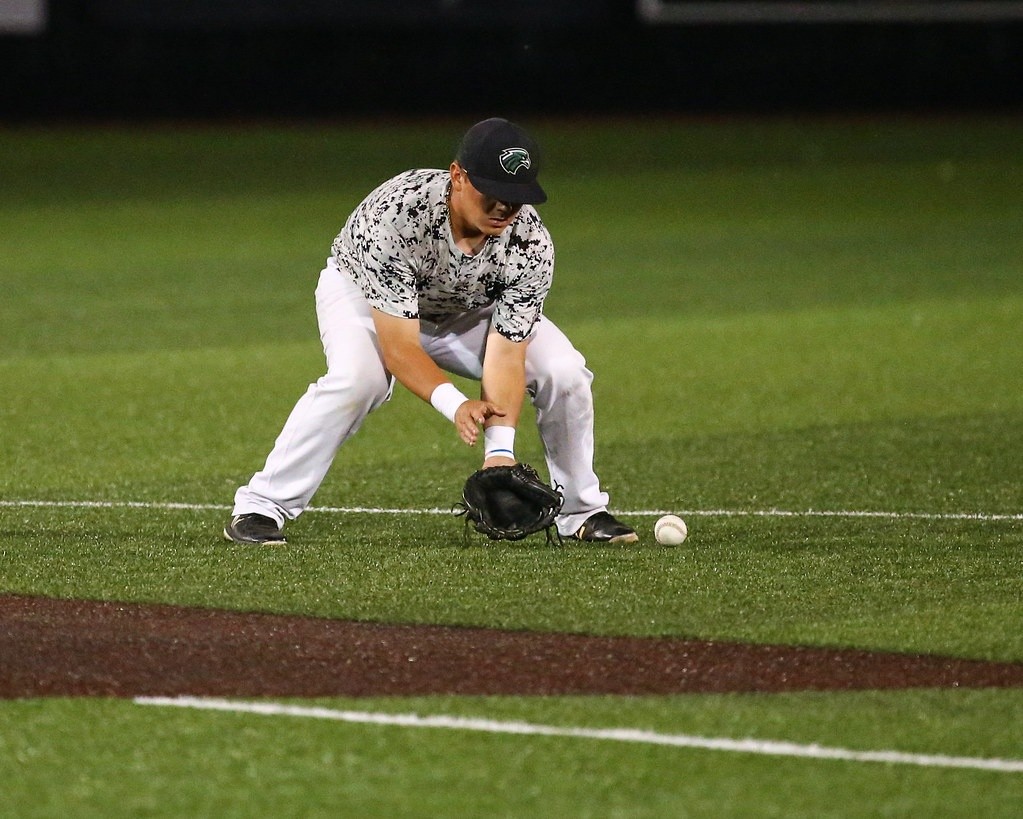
[455,118,547,205]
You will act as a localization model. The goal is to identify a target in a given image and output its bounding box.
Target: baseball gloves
[462,463,565,541]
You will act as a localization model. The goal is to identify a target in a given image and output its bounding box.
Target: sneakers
[223,512,288,546]
[569,511,639,544]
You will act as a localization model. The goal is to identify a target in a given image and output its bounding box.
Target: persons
[224,117,638,544]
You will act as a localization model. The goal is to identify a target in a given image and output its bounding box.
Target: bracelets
[484,425,516,460]
[431,383,469,423]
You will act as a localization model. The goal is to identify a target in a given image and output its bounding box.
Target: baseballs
[654,515,688,547]
